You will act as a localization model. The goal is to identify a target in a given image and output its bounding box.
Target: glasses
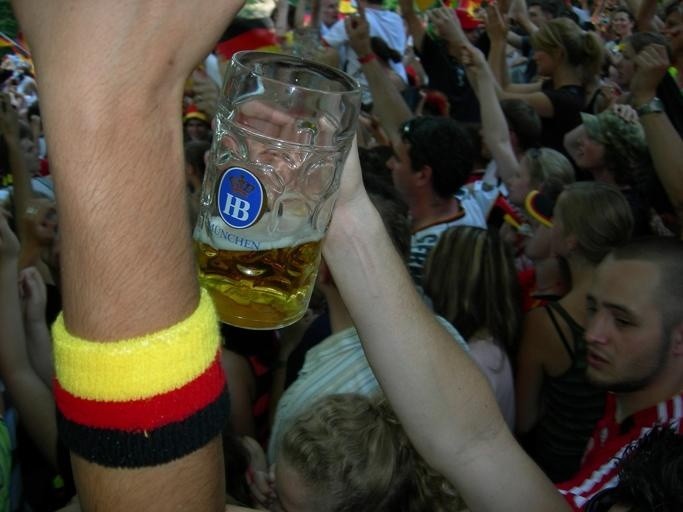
[401,117,426,156]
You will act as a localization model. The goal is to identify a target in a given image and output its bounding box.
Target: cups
[191,49,363,332]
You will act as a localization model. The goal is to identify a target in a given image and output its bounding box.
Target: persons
[0,0,682,511]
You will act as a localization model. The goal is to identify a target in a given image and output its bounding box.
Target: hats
[580,107,650,163]
[525,176,567,229]
